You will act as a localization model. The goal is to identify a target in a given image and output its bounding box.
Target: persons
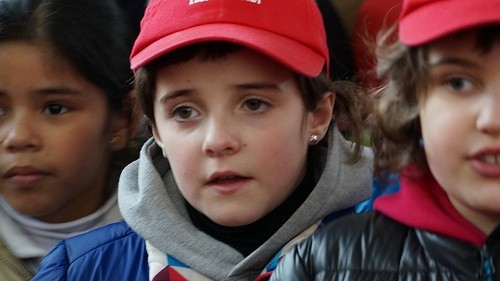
[30,0,399,281]
[269,0,500,281]
[0,0,148,281]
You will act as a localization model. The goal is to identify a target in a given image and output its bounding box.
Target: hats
[398,0,500,45]
[130,0,330,79]
